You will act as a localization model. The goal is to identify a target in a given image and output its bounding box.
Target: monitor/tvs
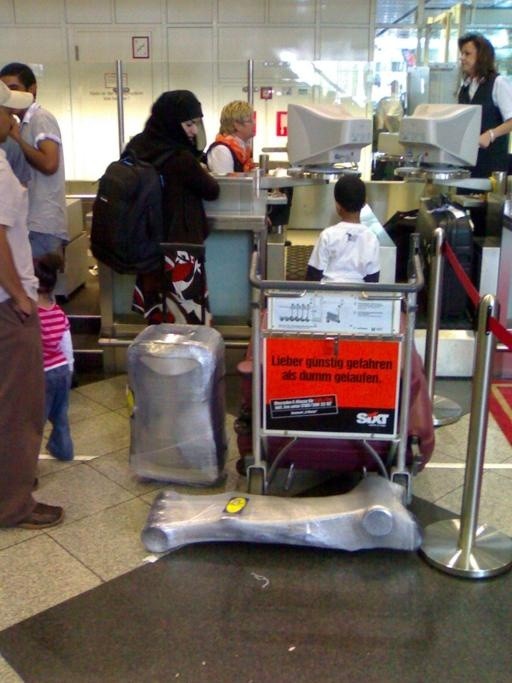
[398,103,483,173]
[287,102,372,174]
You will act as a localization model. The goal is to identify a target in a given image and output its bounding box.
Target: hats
[0,79,34,110]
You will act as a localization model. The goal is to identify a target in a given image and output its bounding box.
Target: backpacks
[90,136,182,275]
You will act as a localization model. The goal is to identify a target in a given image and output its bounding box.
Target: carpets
[2,494,512,680]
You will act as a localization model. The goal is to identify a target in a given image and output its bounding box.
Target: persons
[304,173,382,283]
[455,29,511,201]
[119,87,222,331]
[1,61,71,276]
[31,251,75,460]
[201,98,255,177]
[0,76,68,532]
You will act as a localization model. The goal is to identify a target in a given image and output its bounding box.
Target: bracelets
[488,129,495,143]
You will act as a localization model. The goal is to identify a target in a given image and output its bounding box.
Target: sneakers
[45,442,73,461]
[18,503,64,529]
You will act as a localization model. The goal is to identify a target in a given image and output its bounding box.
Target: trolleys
[239,248,425,507]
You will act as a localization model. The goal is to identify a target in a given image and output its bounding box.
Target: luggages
[244,309,422,418]
[383,201,477,318]
[124,322,228,486]
[236,374,437,474]
